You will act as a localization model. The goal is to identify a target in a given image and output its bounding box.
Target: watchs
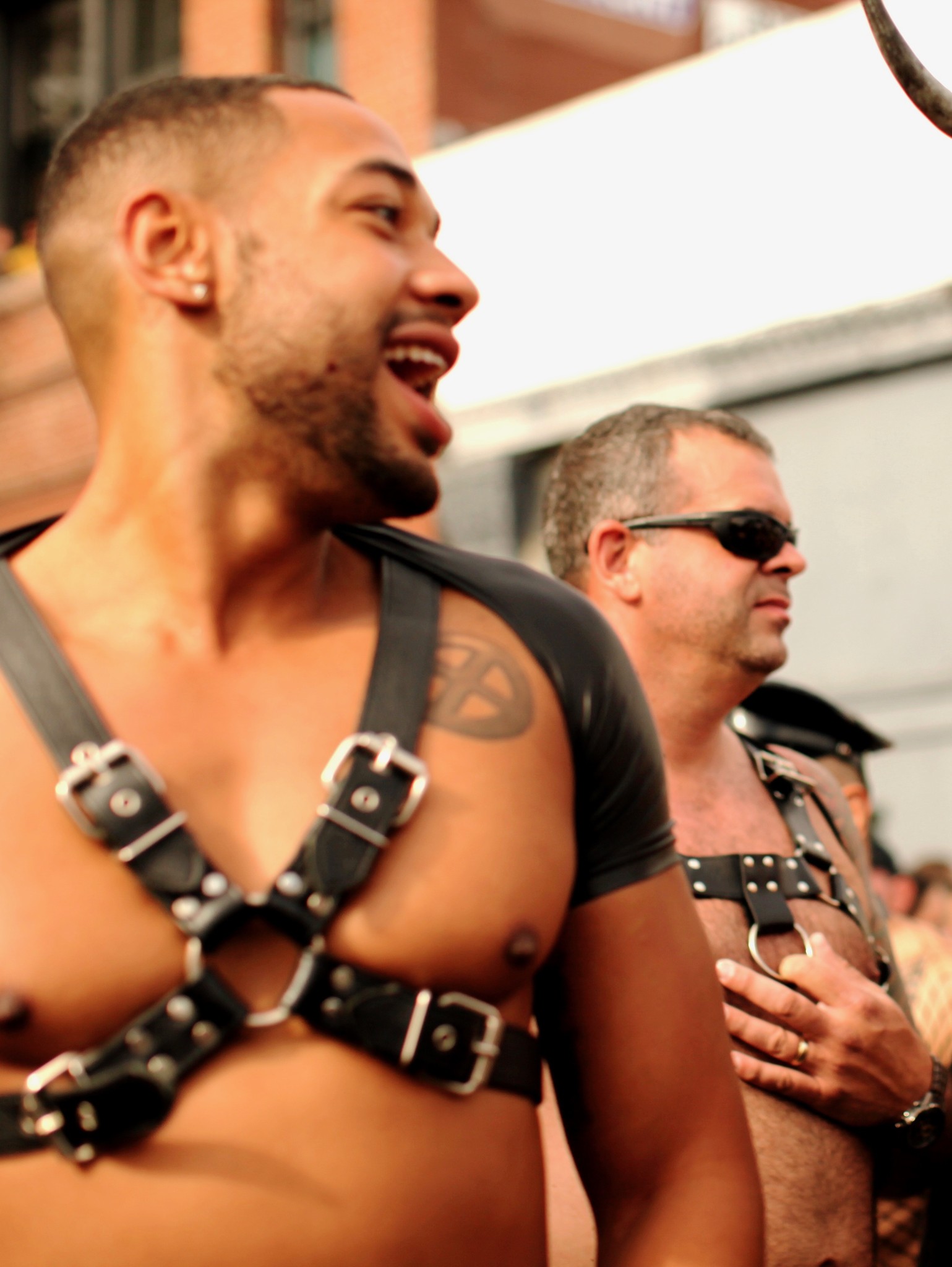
[866,1055,947,1157]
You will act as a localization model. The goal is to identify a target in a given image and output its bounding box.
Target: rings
[789,1037,809,1067]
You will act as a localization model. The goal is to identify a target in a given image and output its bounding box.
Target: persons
[733,676,952,1067]
[0,74,768,1267]
[510,404,952,1267]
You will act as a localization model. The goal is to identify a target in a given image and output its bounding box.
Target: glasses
[583,508,799,562]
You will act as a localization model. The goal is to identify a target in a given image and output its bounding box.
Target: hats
[729,681,892,779]
